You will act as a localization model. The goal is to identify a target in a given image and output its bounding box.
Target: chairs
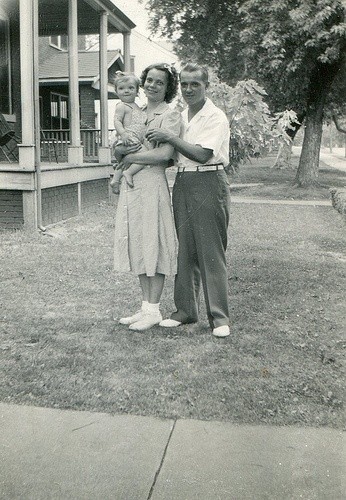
[40,127,58,164]
[0,114,21,163]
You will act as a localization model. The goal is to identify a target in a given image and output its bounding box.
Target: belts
[177,165,224,172]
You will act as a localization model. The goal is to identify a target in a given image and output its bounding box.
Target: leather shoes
[120,310,162,332]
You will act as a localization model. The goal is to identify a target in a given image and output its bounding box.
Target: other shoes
[159,319,181,327]
[213,326,230,337]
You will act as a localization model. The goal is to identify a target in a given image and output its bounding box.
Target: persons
[110,63,183,332]
[145,63,231,338]
[109,72,153,195]
